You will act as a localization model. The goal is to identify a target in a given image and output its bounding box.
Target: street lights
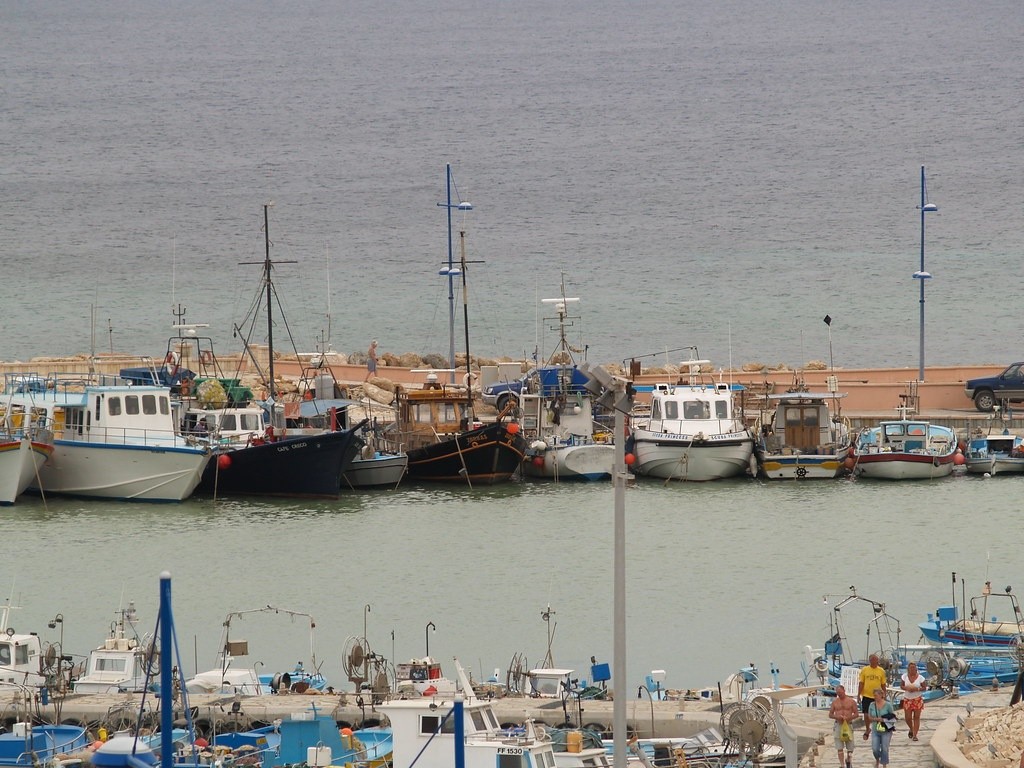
[912,204,938,381]
[597,383,635,768]
[433,203,475,383]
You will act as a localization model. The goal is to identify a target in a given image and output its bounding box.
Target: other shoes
[845,759,852,768]
[913,737,918,741]
[877,722,885,732]
[908,730,913,738]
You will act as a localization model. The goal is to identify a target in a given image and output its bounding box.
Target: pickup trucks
[965,359,1024,410]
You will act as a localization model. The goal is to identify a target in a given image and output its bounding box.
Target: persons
[828,684,859,768]
[856,653,887,740]
[363,339,380,383]
[867,687,898,768]
[899,662,927,742]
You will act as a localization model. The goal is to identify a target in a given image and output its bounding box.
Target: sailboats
[199,198,369,498]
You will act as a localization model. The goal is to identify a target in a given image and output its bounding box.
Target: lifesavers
[202,350,211,367]
[167,351,178,376]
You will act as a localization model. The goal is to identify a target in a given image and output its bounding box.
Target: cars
[481,364,592,416]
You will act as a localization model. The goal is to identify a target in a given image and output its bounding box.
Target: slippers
[863,729,871,740]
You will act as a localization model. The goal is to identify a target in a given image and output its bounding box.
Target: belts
[835,721,852,724]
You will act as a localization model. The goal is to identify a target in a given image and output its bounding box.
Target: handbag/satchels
[840,720,852,742]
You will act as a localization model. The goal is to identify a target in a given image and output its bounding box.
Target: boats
[0,338,331,502]
[341,445,409,487]
[628,345,753,481]
[811,572,1023,710]
[520,267,636,483]
[965,396,1024,477]
[370,372,529,484]
[849,379,964,484]
[753,315,852,479]
[0,572,829,767]
